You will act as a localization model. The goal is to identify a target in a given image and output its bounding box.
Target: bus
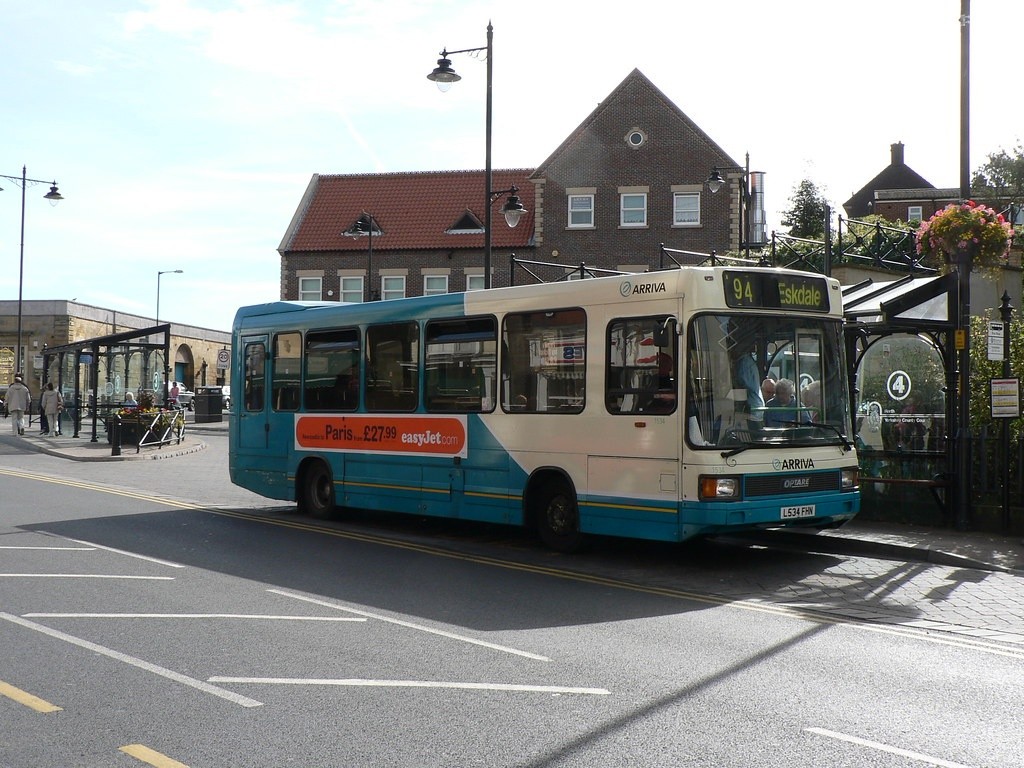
[228,267,871,555]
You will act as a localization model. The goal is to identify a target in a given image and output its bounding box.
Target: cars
[221,385,230,410]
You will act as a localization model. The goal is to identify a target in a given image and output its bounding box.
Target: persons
[169,382,181,409]
[650,353,672,412]
[762,379,820,426]
[4,373,31,435]
[733,334,765,422]
[126,392,137,408]
[36,383,72,437]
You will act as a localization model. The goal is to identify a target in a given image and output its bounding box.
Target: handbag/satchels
[57,402,62,409]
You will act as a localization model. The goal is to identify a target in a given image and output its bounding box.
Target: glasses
[763,389,775,394]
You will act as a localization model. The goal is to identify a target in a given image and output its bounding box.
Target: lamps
[866,201,873,215]
[33,340,38,348]
[42,342,48,351]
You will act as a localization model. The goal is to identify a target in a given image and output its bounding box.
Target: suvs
[138,380,193,412]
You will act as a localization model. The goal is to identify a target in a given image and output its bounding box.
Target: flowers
[916,200,1014,282]
[113,407,185,434]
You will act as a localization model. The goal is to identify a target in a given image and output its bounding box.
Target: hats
[15,377,21,382]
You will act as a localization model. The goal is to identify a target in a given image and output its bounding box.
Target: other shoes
[40,429,45,434]
[55,431,59,435]
[45,432,48,435]
[20,428,24,435]
[48,432,54,437]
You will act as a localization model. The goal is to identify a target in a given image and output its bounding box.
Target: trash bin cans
[195,386,223,424]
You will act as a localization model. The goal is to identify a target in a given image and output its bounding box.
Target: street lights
[342,214,374,302]
[704,149,751,260]
[154,269,184,373]
[0,165,64,374]
[426,20,529,291]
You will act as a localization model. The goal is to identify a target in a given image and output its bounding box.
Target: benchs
[857,477,944,488]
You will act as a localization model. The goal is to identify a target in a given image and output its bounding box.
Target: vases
[942,239,978,265]
[106,416,172,445]
